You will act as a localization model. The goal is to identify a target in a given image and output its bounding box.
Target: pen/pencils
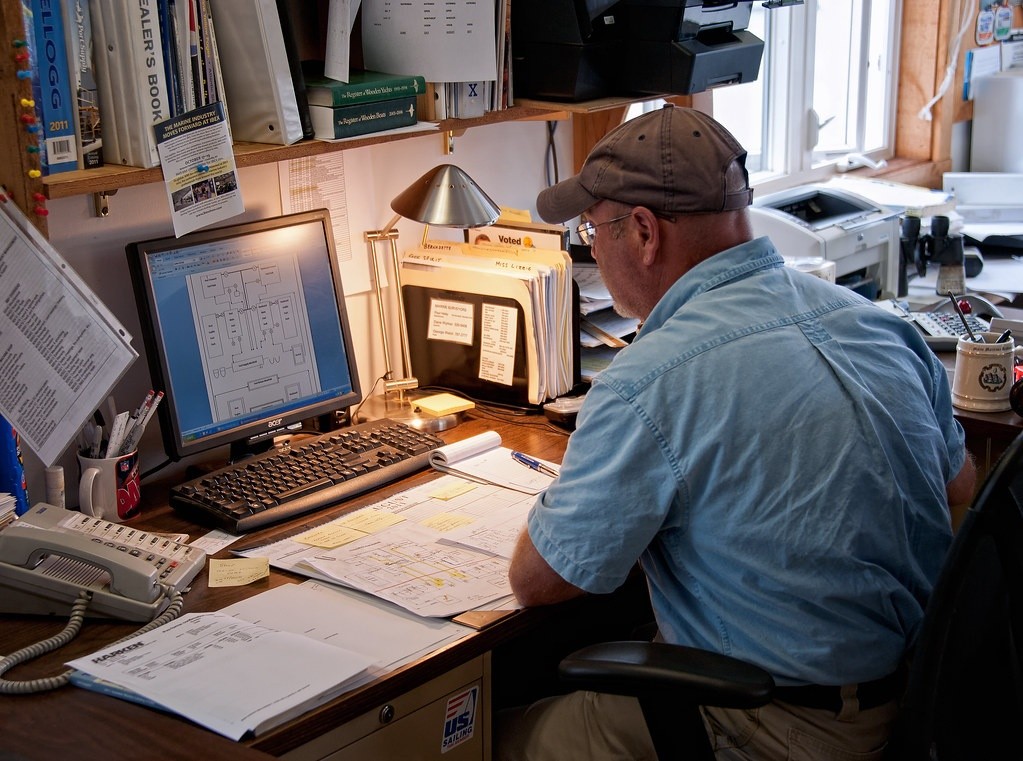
[85,390,161,459]
[997,329,1011,343]
[511,451,557,476]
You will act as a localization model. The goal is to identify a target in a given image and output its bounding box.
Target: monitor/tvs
[125,208,363,466]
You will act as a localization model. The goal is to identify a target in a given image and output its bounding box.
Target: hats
[536,103,754,225]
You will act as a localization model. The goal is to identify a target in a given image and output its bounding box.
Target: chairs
[556,429,1023,761]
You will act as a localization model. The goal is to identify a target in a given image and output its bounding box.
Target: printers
[745,182,900,302]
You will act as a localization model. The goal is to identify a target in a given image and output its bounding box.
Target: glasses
[575,214,676,247]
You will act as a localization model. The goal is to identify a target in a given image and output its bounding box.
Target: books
[18,0,233,176]
[68,671,326,738]
[307,72,427,140]
[464,219,570,253]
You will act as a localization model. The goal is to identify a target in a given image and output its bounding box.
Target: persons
[509,102,978,761]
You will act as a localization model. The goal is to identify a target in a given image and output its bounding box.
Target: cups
[75,447,141,522]
[951,332,1023,411]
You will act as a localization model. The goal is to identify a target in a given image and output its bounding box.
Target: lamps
[362,164,503,434]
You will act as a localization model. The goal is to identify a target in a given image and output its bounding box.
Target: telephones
[0,502,206,624]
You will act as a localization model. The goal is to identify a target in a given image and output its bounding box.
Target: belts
[781,680,886,713]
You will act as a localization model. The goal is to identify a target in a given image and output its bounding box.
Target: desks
[0,351,1023,761]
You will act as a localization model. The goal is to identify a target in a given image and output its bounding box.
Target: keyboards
[168,416,447,532]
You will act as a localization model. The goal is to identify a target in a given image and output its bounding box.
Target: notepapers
[415,393,477,419]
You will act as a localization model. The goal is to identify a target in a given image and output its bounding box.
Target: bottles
[937,233,966,295]
[45,466,64,509]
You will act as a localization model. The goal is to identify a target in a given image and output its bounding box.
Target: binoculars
[898,216,967,297]
